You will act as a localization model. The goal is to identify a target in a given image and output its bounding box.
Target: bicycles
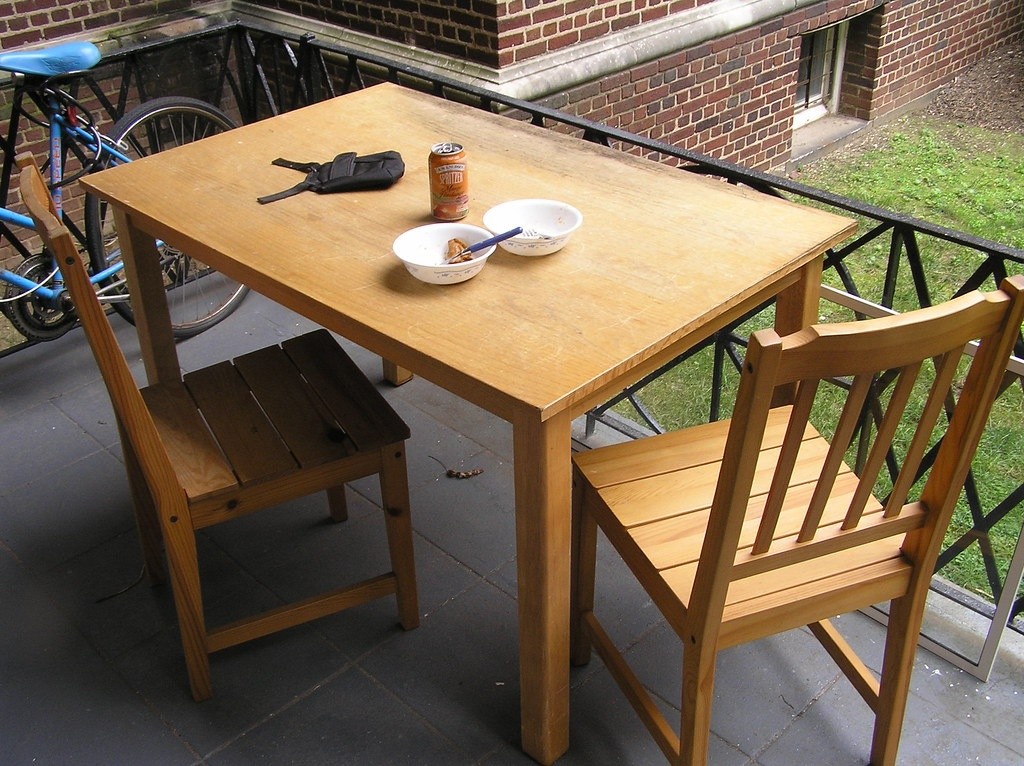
[0,40,252,339]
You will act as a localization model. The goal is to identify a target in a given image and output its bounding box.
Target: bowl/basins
[482,198,583,257]
[392,222,497,285]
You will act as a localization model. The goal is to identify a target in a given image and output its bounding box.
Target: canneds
[428,144,469,221]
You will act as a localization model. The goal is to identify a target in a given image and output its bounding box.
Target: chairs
[20,151,419,699]
[571,274,1023,765]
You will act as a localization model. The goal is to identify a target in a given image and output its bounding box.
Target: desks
[77,82,860,765]
